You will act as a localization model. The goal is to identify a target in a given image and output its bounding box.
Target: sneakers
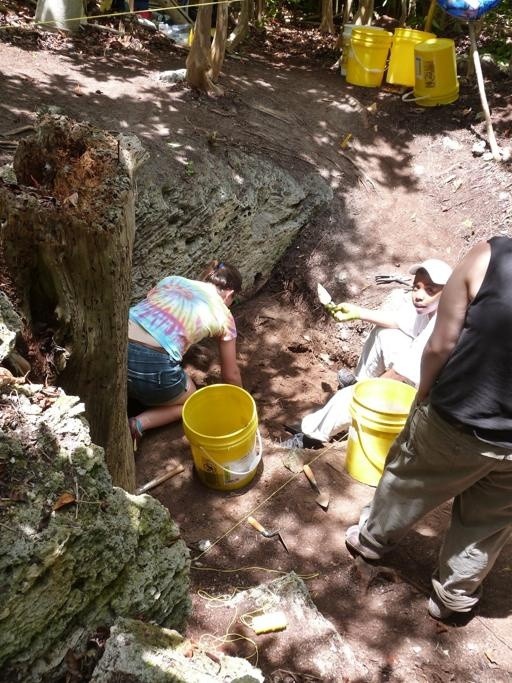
[127,417,143,453]
[337,368,357,388]
[280,433,322,450]
[427,588,455,621]
[345,524,384,561]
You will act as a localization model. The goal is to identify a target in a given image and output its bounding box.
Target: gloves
[327,303,361,322]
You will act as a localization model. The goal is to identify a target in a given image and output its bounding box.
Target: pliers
[375,273,413,286]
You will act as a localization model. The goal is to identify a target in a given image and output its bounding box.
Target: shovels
[318,282,343,320]
[248,517,289,553]
[303,464,329,509]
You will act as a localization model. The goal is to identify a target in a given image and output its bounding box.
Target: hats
[408,258,453,285]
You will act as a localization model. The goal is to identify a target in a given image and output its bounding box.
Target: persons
[125,256,247,440]
[344,237,511,620]
[325,256,453,389]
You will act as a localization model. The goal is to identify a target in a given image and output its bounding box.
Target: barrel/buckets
[181,383,264,491]
[402,37,460,106]
[345,377,416,488]
[341,24,392,90]
[386,26,437,88]
